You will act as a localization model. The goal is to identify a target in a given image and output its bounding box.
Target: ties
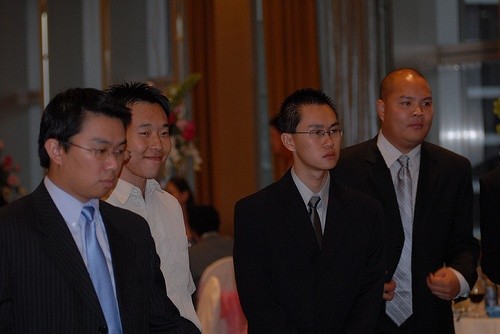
[307,196,323,251]
[386,154,414,327]
[79,206,122,334]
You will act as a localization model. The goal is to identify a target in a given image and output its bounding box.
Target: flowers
[152,75,205,169]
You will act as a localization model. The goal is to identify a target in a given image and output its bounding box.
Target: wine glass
[468,280,486,319]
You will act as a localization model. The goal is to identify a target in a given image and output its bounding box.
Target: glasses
[65,139,132,161]
[293,128,344,138]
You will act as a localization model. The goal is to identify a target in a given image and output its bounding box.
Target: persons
[335,68,481,334]
[481,167,500,282]
[163,177,234,283]
[96,82,201,332]
[0,87,202,334]
[233,87,388,334]
